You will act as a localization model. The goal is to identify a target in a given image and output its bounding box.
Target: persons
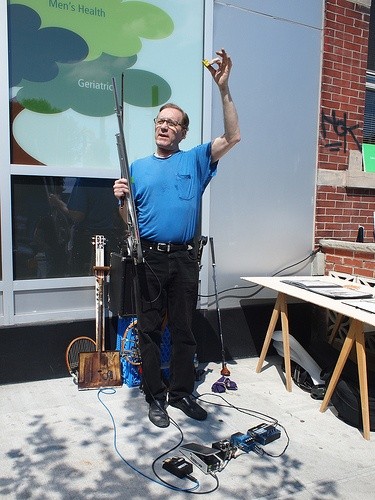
[47,177,117,277]
[112,49,241,427]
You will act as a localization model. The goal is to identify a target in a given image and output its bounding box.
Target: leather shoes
[168,395,208,421]
[148,399,170,427]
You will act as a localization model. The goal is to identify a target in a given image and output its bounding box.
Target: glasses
[153,117,186,129]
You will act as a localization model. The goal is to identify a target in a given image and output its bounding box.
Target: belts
[142,241,188,253]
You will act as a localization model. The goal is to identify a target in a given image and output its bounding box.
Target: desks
[239,274,375,441]
